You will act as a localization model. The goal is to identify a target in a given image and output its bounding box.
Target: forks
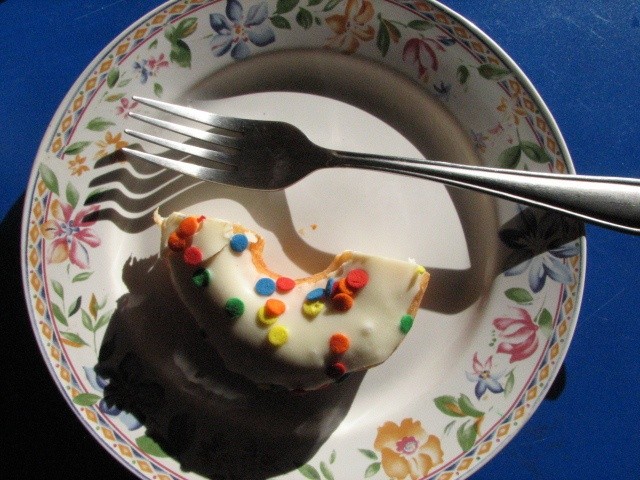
[121,95,639,237]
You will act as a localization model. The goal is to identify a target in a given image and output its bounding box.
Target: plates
[21,0,588,479]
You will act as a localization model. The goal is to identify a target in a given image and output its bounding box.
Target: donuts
[152,206,431,396]
